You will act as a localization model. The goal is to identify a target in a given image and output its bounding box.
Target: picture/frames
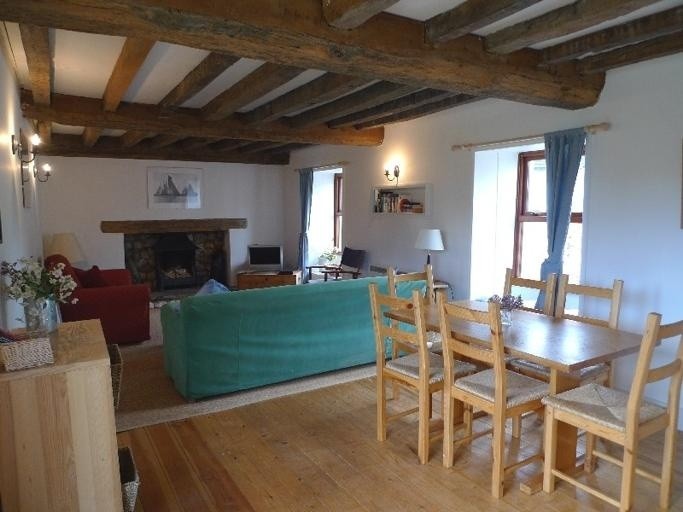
[146,165,204,210]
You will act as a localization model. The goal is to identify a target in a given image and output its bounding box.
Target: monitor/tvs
[247,245,284,271]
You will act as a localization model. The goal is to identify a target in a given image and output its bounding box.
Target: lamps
[10,126,41,163]
[33,161,51,183]
[413,227,446,263]
[383,161,399,182]
[44,232,86,264]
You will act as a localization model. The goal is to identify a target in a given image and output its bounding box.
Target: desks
[0,317,124,512]
[237,270,302,290]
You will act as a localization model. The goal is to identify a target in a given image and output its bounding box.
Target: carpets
[115,307,378,435]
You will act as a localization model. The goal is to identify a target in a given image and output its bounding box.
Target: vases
[22,298,42,340]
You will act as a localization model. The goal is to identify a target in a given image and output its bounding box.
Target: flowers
[0,257,79,309]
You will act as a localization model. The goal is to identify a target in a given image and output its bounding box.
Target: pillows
[193,279,231,297]
[78,264,110,288]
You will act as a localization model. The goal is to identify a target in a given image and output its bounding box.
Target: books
[374,191,422,214]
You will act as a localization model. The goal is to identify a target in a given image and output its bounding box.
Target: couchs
[160,274,428,403]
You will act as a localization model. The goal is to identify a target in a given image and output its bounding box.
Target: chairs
[305,246,366,282]
[44,254,151,346]
